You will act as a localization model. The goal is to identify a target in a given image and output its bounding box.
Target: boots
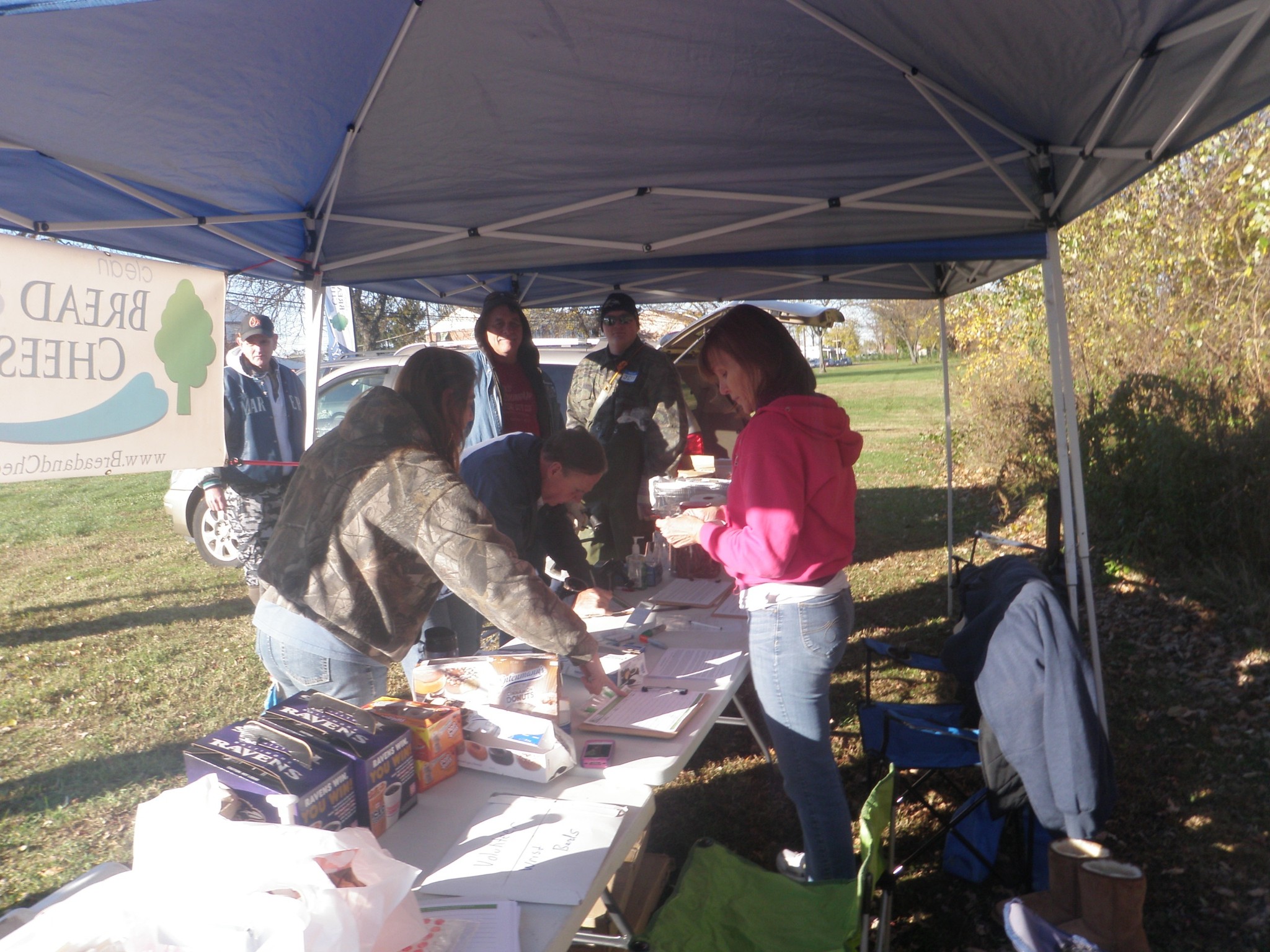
[996,839,1109,929]
[1058,860,1149,952]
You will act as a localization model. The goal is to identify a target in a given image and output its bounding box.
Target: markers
[639,635,667,649]
[641,624,666,636]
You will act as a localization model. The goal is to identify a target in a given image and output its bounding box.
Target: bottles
[675,501,721,580]
[424,626,460,660]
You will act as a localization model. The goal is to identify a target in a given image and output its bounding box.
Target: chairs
[858,537,1069,952]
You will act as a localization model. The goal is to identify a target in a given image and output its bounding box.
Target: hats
[600,293,636,317]
[239,314,274,342]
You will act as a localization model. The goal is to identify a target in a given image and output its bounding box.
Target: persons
[250,347,626,710]
[195,313,306,606]
[564,293,687,581]
[654,305,864,883]
[452,291,588,648]
[400,426,607,702]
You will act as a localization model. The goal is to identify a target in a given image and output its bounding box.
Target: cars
[808,357,854,368]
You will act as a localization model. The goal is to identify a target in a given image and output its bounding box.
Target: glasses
[602,313,634,326]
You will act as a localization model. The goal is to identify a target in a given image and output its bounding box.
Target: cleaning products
[625,535,649,591]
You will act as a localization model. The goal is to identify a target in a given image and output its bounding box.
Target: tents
[0,0,1270,737]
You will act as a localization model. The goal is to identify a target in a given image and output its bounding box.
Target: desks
[0,561,772,952]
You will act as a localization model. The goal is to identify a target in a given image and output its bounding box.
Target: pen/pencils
[688,620,722,630]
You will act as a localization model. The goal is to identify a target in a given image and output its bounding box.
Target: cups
[308,819,341,832]
[368,780,386,834]
[383,782,402,829]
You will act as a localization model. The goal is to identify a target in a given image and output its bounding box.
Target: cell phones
[580,739,614,768]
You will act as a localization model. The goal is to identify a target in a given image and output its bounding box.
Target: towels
[975,580,1107,842]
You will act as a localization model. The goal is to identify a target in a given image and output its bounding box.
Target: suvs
[159,301,851,569]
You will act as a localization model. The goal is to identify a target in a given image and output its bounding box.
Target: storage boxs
[184,719,358,832]
[422,694,577,784]
[415,747,459,793]
[260,689,417,839]
[361,696,464,761]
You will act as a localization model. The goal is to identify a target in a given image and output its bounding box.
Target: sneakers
[776,849,814,882]
